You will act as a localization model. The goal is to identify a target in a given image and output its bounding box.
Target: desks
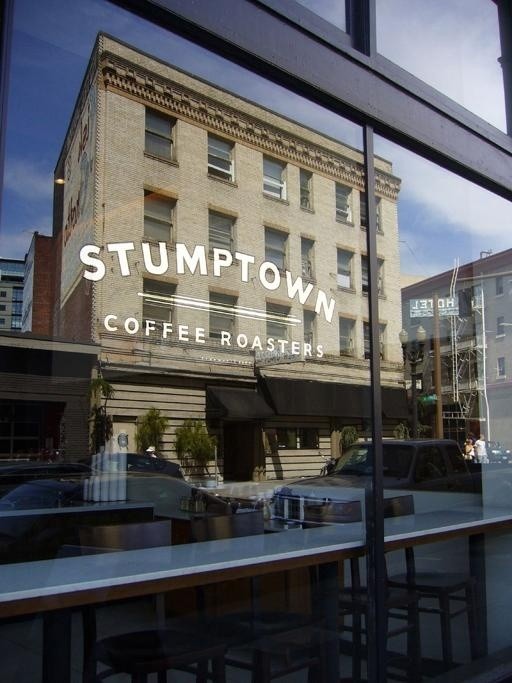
[0,508,512,682]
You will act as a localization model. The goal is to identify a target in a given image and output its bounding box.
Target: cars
[0,452,265,566]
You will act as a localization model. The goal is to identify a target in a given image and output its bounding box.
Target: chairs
[301,501,418,682]
[186,510,330,683]
[381,494,477,682]
[77,520,229,683]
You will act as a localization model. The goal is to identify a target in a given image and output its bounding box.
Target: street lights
[399,326,426,439]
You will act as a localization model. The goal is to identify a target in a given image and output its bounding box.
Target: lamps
[54,155,65,184]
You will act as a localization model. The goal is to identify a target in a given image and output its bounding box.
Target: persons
[145,445,157,458]
[39,447,64,464]
[463,432,488,464]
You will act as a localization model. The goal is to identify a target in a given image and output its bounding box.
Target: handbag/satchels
[464,455,472,460]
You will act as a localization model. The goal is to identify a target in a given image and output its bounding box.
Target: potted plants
[172,417,224,488]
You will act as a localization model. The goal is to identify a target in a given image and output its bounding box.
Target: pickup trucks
[254,440,512,533]
